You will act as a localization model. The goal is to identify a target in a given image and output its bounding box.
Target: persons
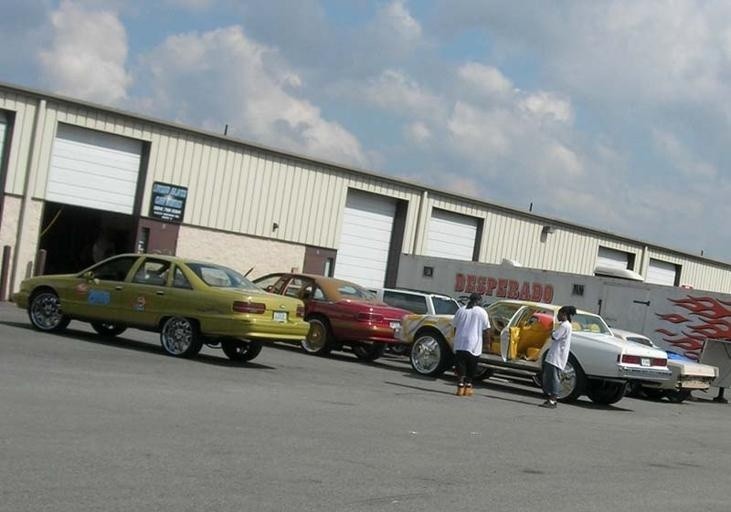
[540,306,576,407]
[451,294,492,396]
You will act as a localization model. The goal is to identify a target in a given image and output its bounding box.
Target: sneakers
[542,400,557,409]
[456,387,473,397]
